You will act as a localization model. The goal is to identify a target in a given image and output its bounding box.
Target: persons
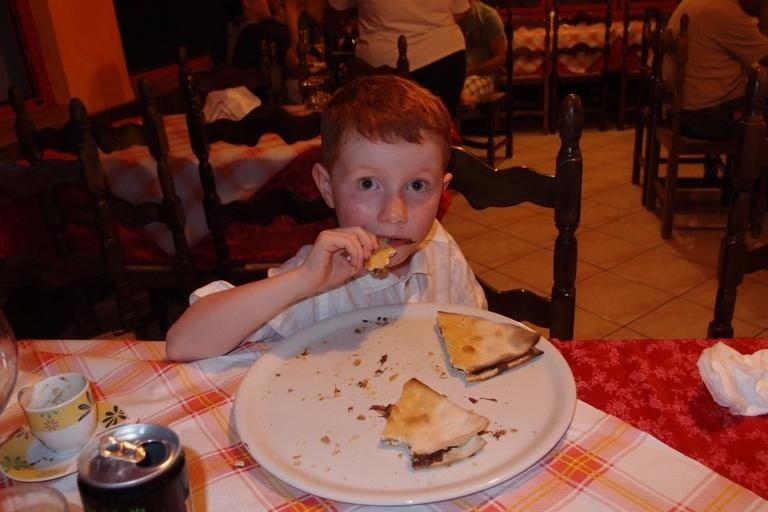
[328,0,509,224]
[658,0,768,246]
[231,20,291,106]
[163,70,491,362]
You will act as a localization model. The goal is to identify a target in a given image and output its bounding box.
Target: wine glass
[301,80,324,112]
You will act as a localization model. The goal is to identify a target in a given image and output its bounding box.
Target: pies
[436,311,545,384]
[345,238,397,278]
[380,376,492,470]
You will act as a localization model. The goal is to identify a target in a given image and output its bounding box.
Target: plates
[232,301,579,507]
[0,402,129,482]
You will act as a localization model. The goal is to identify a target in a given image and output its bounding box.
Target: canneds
[76,421,191,512]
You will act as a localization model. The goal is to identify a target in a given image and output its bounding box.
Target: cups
[18,372,97,457]
